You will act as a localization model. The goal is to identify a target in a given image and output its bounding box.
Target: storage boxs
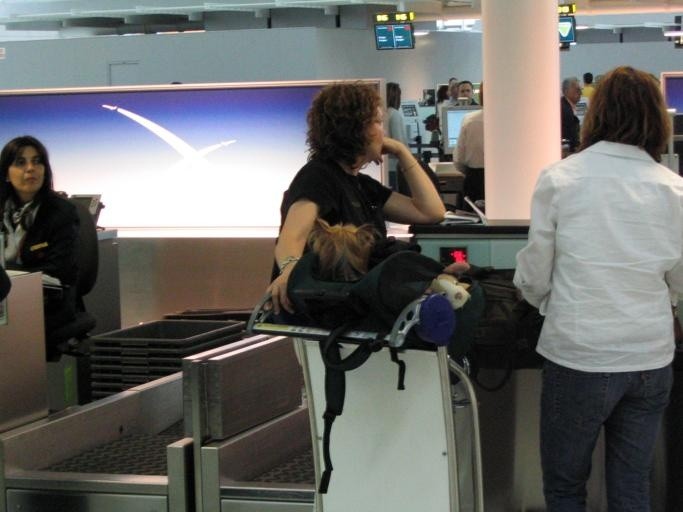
[84,302,268,406]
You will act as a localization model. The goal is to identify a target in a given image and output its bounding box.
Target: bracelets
[399,159,418,172]
[277,254,300,272]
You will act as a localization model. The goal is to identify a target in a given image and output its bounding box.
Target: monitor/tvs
[400,101,422,121]
[574,97,589,124]
[373,23,415,51]
[471,82,481,103]
[660,71,683,114]
[441,106,482,157]
[559,16,576,43]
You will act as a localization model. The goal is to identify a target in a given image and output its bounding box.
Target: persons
[0,136,100,364]
[515,65,682,512]
[377,72,599,220]
[264,82,448,316]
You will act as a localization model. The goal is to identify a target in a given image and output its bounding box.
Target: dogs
[312,217,375,284]
